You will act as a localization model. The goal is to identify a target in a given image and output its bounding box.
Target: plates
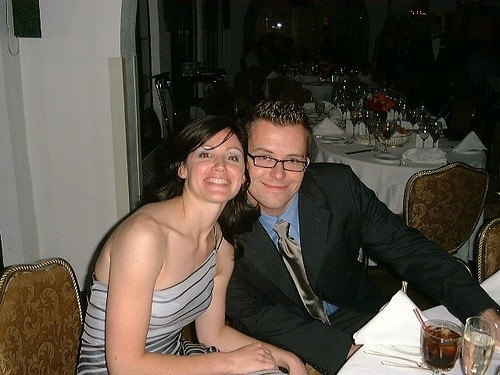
[401,147,446,163]
[372,153,401,164]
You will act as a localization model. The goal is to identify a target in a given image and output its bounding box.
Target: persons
[372,17,403,87]
[415,14,456,115]
[397,15,414,58]
[76,115,309,375]
[221,97,500,375]
[245,31,356,74]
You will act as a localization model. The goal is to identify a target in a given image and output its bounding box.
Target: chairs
[473,217,500,283]
[0,257,84,375]
[404,163,489,256]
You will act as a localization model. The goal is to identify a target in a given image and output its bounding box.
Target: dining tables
[301,101,431,136]
[335,270,500,375]
[265,72,372,101]
[309,131,487,266]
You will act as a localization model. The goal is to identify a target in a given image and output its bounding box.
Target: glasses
[247,152,308,172]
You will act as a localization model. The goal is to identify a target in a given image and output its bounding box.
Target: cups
[420,319,464,373]
[461,316,496,375]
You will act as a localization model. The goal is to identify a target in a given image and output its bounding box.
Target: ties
[273,220,332,326]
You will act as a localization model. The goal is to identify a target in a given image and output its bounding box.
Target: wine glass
[331,81,443,153]
